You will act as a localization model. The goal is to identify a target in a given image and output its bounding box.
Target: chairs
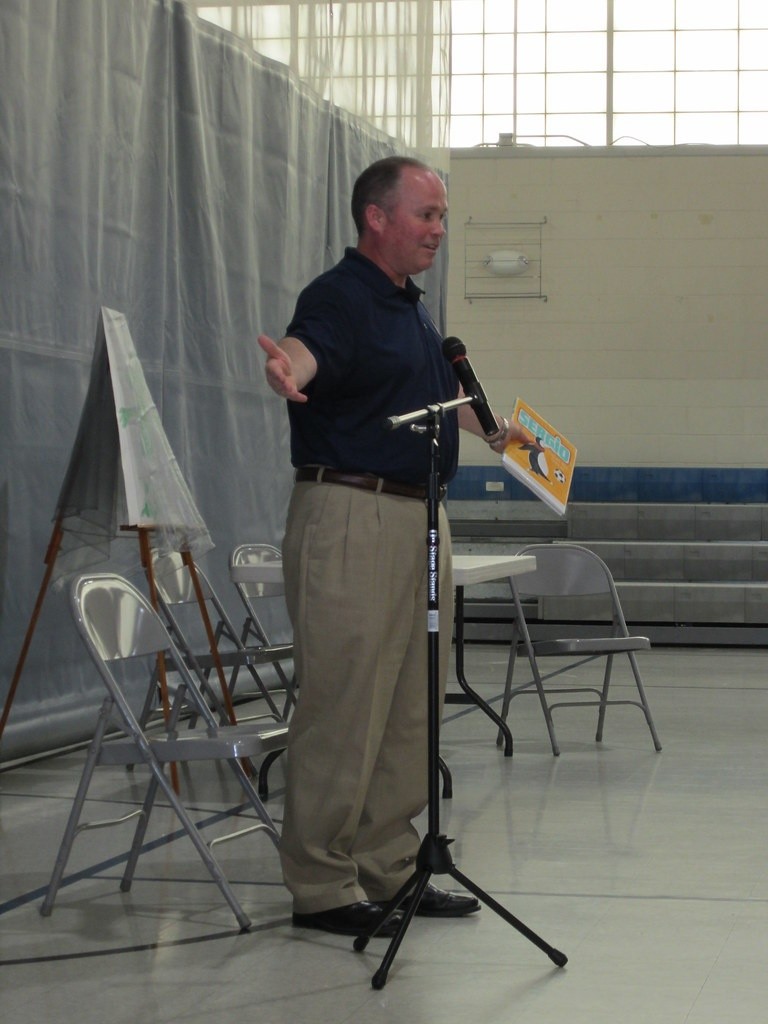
[39,573,292,932]
[216,543,297,726]
[495,544,663,757]
[124,545,295,778]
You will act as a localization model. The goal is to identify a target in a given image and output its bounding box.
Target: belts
[294,465,447,501]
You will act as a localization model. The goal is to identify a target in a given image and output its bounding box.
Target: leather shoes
[292,900,405,939]
[374,884,481,917]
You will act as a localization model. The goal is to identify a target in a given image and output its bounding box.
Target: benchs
[444,465,768,624]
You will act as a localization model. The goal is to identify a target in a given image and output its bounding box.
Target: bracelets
[484,416,509,446]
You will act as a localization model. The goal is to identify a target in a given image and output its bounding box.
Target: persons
[257,157,544,937]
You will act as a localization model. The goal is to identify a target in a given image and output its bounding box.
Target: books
[501,397,578,516]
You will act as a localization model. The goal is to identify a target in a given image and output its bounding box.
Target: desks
[228,554,537,798]
